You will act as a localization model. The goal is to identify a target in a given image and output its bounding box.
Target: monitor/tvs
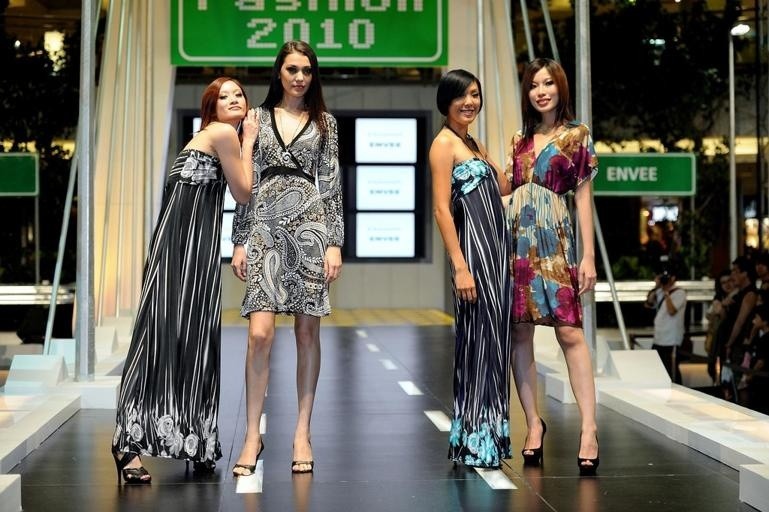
[651,204,680,223]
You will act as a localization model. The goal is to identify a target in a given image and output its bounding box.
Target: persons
[111,75,262,484]
[643,224,769,413]
[486,56,602,476]
[229,38,347,478]
[427,68,515,471]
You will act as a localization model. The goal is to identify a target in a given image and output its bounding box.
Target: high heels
[111,445,152,488]
[577,428,600,476]
[185,460,216,476]
[291,437,314,473]
[232,436,265,477]
[522,418,547,467]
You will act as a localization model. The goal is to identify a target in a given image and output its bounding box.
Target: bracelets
[490,159,495,168]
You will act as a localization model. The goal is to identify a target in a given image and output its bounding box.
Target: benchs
[629,331,713,388]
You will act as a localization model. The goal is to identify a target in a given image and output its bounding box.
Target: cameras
[658,271,671,284]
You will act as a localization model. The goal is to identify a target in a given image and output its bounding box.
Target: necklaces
[278,104,306,164]
[538,121,558,135]
